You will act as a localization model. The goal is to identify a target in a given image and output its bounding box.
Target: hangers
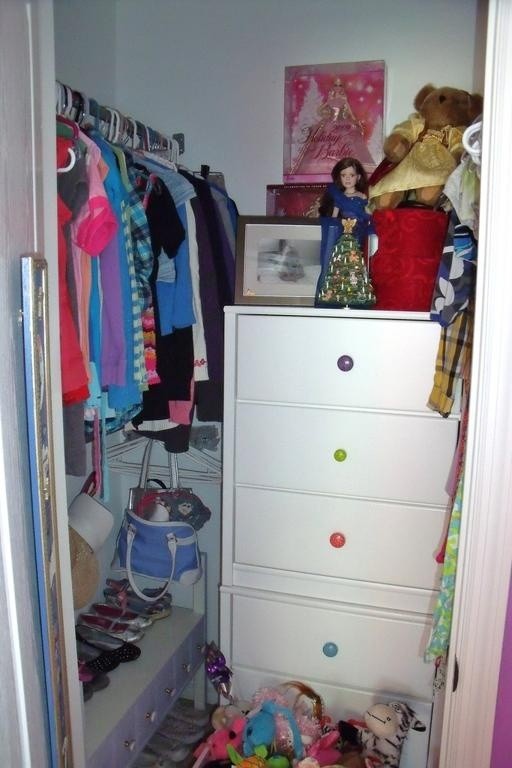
[53,80,224,182]
[100,435,223,486]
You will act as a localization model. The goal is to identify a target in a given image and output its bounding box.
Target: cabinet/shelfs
[218,304,463,723]
[86,605,207,768]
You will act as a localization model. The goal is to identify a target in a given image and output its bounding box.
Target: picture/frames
[235,216,322,308]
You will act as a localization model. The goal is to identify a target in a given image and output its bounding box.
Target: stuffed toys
[366,82,483,211]
[192,680,426,768]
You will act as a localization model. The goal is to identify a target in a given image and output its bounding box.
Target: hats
[69,525,100,608]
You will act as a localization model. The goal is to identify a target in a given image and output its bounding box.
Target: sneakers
[76,578,171,702]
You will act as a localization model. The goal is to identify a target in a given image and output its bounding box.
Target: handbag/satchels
[117,480,210,585]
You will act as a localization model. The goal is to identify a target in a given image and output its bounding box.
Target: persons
[310,156,380,307]
[287,75,375,172]
